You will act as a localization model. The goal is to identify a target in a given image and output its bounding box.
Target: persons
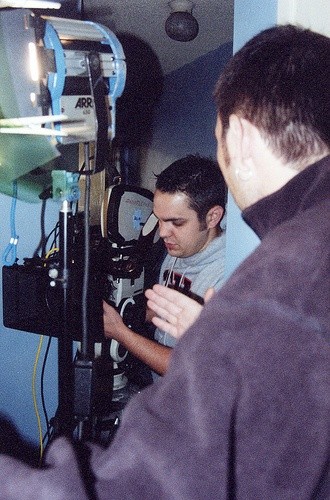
[97,151,226,376]
[0,24,330,499]
